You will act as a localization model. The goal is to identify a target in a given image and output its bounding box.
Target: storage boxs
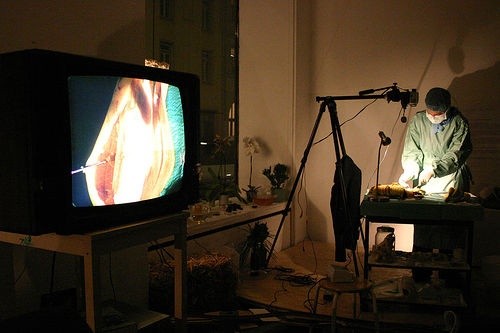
[100,306,170,333]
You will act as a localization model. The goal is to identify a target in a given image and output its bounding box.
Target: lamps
[370,131,391,201]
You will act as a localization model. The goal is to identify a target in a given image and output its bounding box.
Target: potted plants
[262,164,290,202]
[198,167,241,206]
[238,221,280,270]
[241,185,261,203]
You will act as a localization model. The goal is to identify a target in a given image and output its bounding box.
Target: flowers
[242,135,265,185]
[207,134,226,193]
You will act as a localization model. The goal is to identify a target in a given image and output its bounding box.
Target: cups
[190,204,211,223]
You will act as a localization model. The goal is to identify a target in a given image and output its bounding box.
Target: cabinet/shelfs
[360,198,484,315]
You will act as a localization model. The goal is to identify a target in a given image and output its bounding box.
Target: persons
[398,87,474,282]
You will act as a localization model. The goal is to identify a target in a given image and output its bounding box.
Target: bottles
[374,227,395,262]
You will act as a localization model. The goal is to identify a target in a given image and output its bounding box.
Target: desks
[100,201,292,303]
[0,213,189,333]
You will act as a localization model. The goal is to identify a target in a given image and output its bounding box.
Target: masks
[425,110,447,124]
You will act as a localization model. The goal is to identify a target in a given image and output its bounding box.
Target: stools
[310,275,380,333]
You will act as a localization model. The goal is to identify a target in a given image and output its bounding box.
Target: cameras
[409,88,419,106]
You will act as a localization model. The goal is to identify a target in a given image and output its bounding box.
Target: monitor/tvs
[0,49,199,234]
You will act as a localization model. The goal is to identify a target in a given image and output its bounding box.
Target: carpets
[169,297,450,333]
